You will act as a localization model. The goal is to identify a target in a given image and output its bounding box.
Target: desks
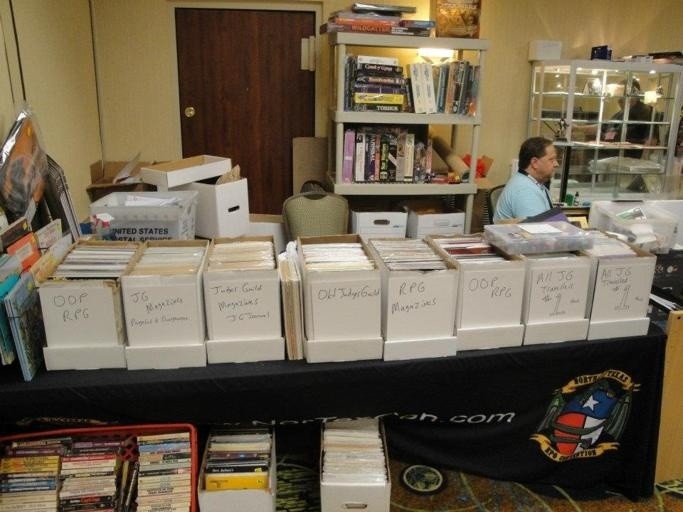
[650,276,683,493]
[1,326,669,503]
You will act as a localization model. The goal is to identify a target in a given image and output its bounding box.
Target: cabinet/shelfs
[328,30,489,235]
[527,58,683,201]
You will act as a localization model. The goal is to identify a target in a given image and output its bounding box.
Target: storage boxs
[429,235,524,349]
[202,237,286,364]
[86,159,153,202]
[319,417,393,511]
[527,38,562,61]
[367,237,457,359]
[404,206,465,236]
[581,228,655,340]
[348,202,409,237]
[140,153,251,242]
[39,239,146,372]
[297,234,382,362]
[121,240,210,370]
[521,250,592,344]
[196,427,278,512]
[587,193,680,255]
[245,213,290,269]
[90,190,200,243]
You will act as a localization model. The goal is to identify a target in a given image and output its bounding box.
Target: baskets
[0,423,197,512]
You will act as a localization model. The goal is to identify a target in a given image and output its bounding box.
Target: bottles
[573,191,580,206]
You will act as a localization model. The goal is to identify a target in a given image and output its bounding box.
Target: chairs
[488,183,506,225]
[282,191,349,242]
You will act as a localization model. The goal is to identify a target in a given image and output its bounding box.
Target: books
[322,420,388,488]
[279,238,304,361]
[302,231,637,272]
[205,433,272,491]
[0,216,281,382]
[319,3,435,37]
[0,432,193,512]
[344,127,433,184]
[343,53,479,117]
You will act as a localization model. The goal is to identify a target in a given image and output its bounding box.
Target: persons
[491,136,559,224]
[571,78,658,183]
